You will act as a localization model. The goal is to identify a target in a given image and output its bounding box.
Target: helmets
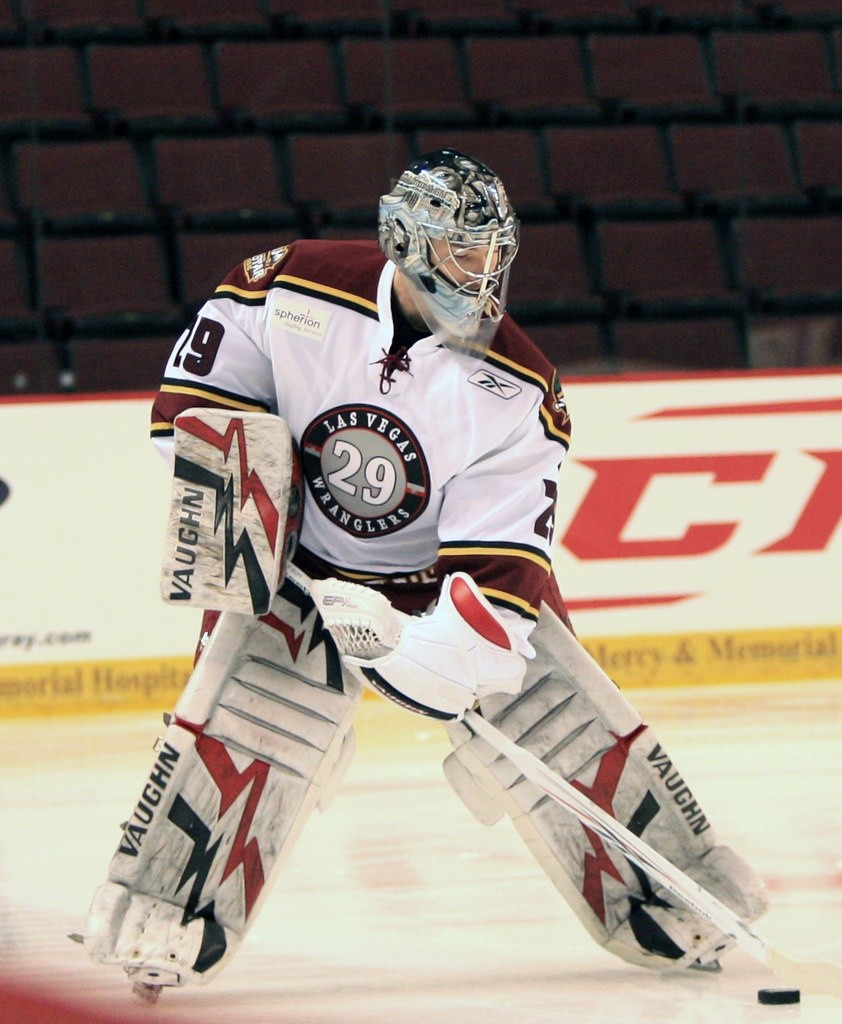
[378,150,521,366]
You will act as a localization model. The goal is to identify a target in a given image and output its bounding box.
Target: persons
[86,150,766,1004]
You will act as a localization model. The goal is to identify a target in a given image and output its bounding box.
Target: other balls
[755,988,801,1006]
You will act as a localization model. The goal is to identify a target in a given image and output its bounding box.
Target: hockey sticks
[269,541,841,1002]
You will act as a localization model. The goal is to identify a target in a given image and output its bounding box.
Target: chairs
[0,0,842,394]
